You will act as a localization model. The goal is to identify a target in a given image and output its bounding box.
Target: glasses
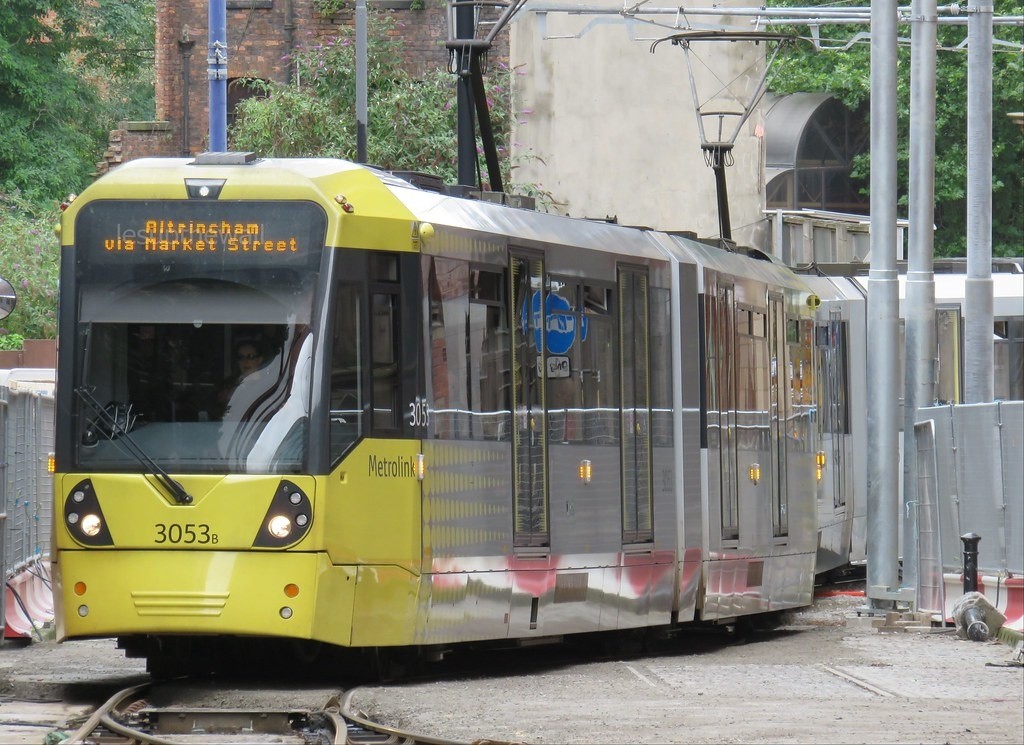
[237,353,260,360]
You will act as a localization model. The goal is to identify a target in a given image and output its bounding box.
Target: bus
[46,152,1023,685]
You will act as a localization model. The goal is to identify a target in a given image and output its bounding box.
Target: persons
[208,335,268,416]
